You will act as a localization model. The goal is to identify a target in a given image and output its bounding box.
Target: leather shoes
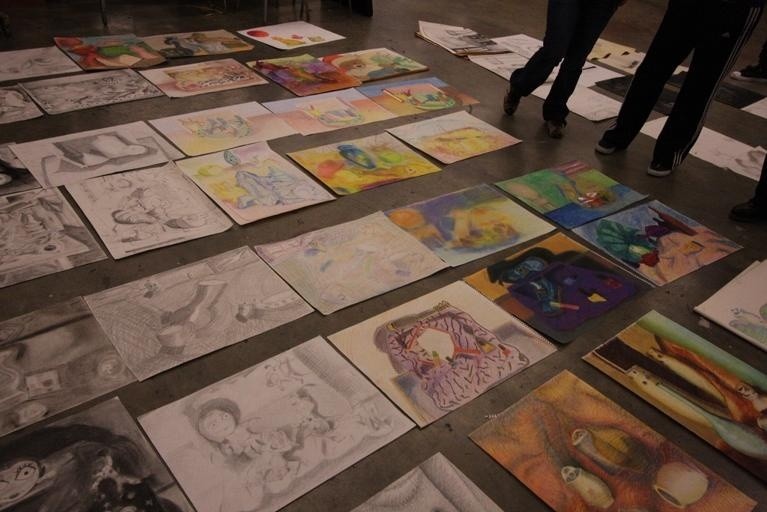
[727,201,767,222]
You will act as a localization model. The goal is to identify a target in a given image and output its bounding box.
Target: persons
[730,43,767,82]
[729,154,767,222]
[595,1,766,177]
[503,1,621,138]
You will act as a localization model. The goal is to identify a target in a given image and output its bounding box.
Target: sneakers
[647,157,672,178]
[547,119,566,139]
[502,77,518,116]
[595,137,628,156]
[729,65,765,83]
